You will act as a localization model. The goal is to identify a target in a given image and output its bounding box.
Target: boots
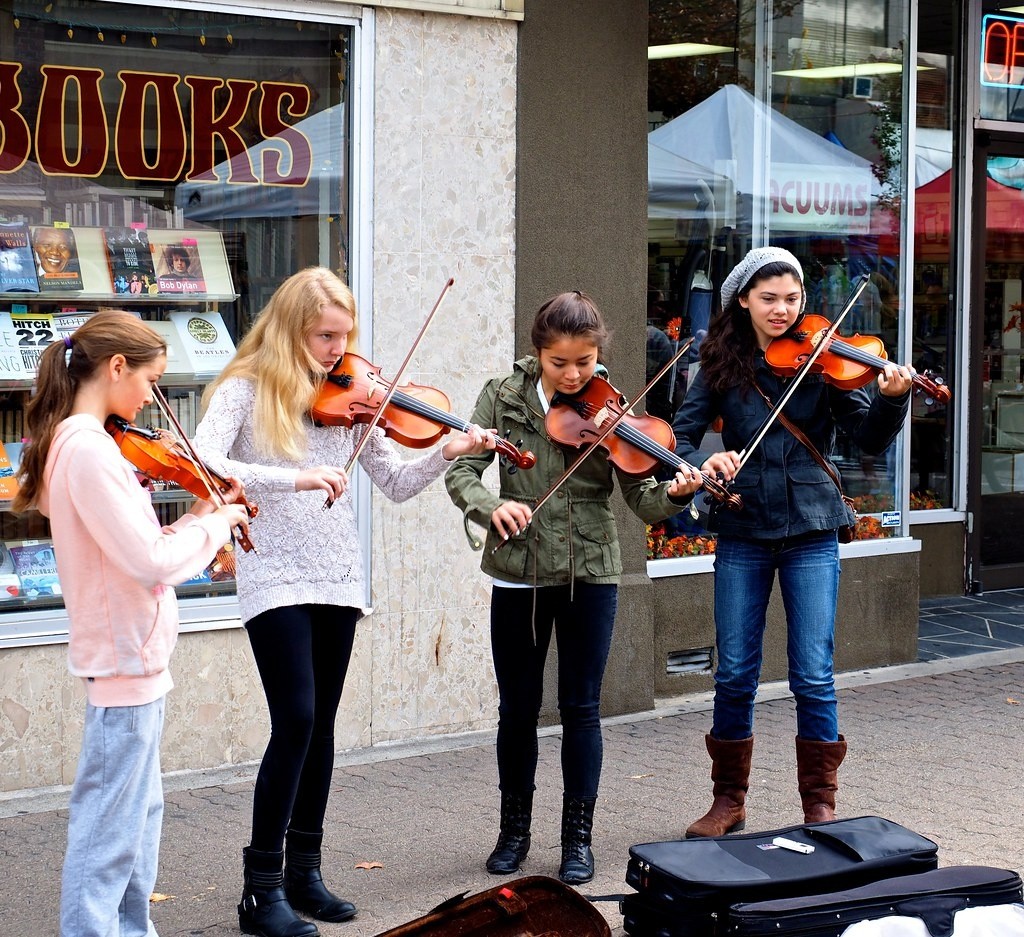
[486,784,535,873]
[558,792,599,882]
[794,731,847,825]
[237,845,322,937]
[283,826,358,921]
[684,734,756,838]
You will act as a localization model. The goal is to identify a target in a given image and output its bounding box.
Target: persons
[159,248,198,278]
[33,227,84,291]
[661,245,915,839]
[104,228,158,294]
[444,291,704,885]
[190,265,498,937]
[13,311,249,937]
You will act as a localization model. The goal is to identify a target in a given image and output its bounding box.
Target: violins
[764,313,952,405]
[105,413,259,518]
[545,375,745,511]
[305,351,537,474]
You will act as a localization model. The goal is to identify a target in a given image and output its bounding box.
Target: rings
[686,479,692,482]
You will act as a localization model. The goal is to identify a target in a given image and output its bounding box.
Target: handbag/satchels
[839,495,861,544]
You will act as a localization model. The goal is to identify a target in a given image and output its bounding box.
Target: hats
[720,246,806,319]
